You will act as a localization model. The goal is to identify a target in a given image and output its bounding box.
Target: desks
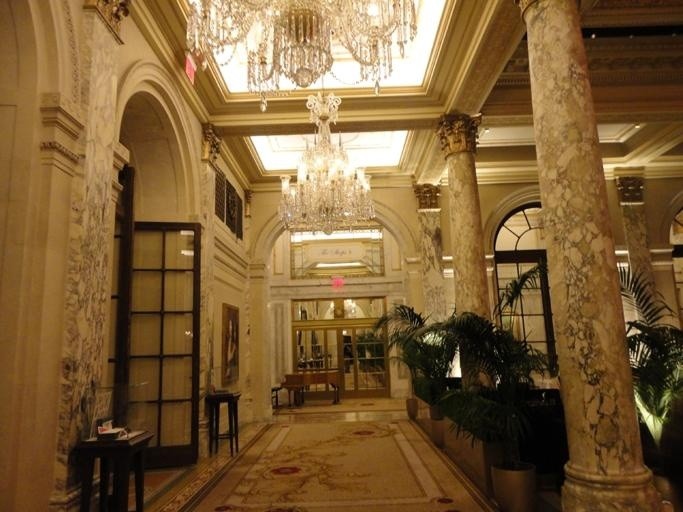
[73,429,156,511]
[207,391,243,457]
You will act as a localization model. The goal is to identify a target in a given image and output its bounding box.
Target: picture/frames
[221,302,240,387]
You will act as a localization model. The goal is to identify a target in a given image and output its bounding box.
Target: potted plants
[371,302,553,511]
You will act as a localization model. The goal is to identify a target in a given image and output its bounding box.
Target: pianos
[283,369,344,406]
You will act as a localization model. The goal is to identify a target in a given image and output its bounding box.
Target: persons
[225,318,237,368]
[343,343,353,374]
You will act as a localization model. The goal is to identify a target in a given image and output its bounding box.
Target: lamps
[186,1,421,113]
[278,91,376,234]
